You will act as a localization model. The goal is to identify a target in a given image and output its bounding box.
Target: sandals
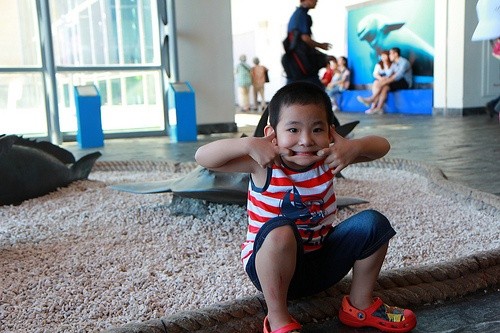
[338,294,417,333]
[263,311,302,333]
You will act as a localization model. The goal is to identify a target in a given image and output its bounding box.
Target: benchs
[341,88,433,116]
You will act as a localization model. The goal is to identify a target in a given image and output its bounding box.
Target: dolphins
[470,0,500,61]
[356,13,434,77]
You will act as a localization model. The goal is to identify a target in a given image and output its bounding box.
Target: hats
[471,0,500,41]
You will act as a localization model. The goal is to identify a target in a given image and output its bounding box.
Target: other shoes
[345,132,354,139]
[365,109,373,114]
[369,108,383,115]
[487,101,495,118]
[358,96,370,107]
[332,105,339,111]
[241,107,267,111]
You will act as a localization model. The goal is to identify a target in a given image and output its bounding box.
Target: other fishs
[0,132,103,206]
[110,103,370,208]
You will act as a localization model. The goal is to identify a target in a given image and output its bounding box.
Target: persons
[357,47,412,114]
[321,55,350,112]
[287,0,355,140]
[195,81,416,333]
[236,54,266,111]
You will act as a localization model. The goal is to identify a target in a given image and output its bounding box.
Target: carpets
[0,158,500,332]
